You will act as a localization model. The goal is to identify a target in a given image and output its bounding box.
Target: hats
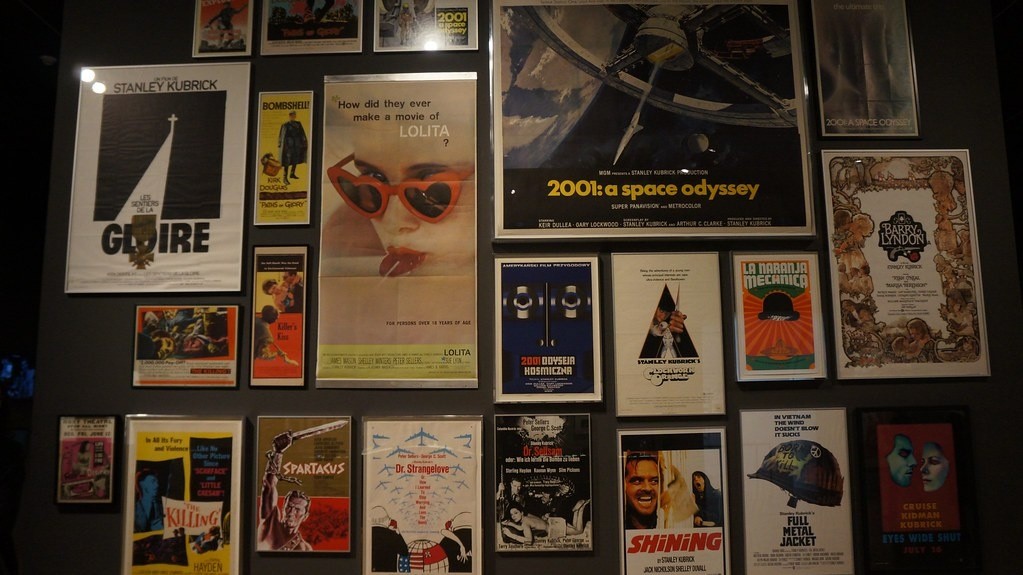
[288,109,297,115]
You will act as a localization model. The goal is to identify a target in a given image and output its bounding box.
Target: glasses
[325,152,474,225]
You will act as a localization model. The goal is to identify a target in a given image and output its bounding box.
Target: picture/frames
[56,0,995,575]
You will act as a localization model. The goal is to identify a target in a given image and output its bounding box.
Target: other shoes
[284,178,289,185]
[290,174,299,179]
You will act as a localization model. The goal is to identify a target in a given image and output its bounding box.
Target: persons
[399,3,412,45]
[263,271,303,313]
[77,441,90,473]
[886,435,948,491]
[625,454,659,528]
[691,472,722,527]
[649,299,687,338]
[328,113,475,276]
[303,0,335,24]
[496,478,591,545]
[277,110,308,184]
[205,2,247,46]
